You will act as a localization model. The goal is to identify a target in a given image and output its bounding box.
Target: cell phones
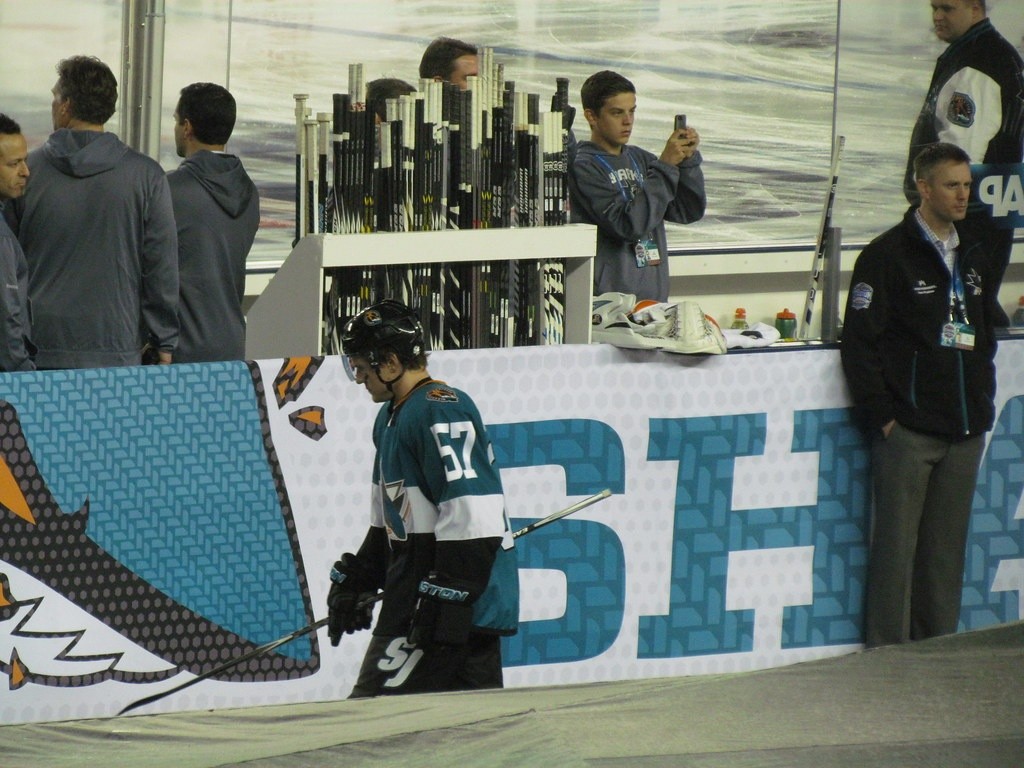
[673,114,686,138]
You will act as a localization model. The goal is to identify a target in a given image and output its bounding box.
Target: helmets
[339,300,424,383]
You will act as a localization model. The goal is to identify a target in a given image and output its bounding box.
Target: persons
[1,49,268,372]
[326,298,520,700]
[839,144,1004,656]
[570,70,713,302]
[364,76,418,123]
[419,36,481,91]
[906,1,1023,300]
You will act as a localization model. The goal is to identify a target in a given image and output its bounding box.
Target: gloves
[379,580,471,679]
[326,554,383,647]
